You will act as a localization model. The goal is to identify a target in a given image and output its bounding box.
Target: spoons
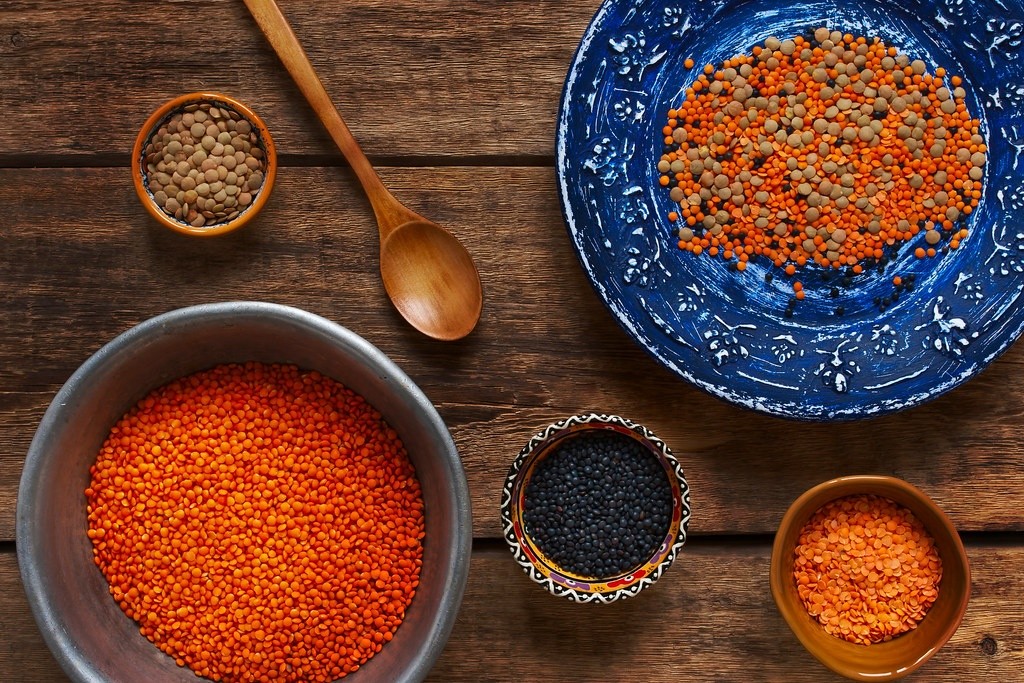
[243,0,483,342]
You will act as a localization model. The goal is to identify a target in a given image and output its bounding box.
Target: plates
[555,0,1024,426]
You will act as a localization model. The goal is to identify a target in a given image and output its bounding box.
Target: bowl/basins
[769,473,971,682]
[499,413,692,605]
[14,300,473,683]
[132,91,279,237]
